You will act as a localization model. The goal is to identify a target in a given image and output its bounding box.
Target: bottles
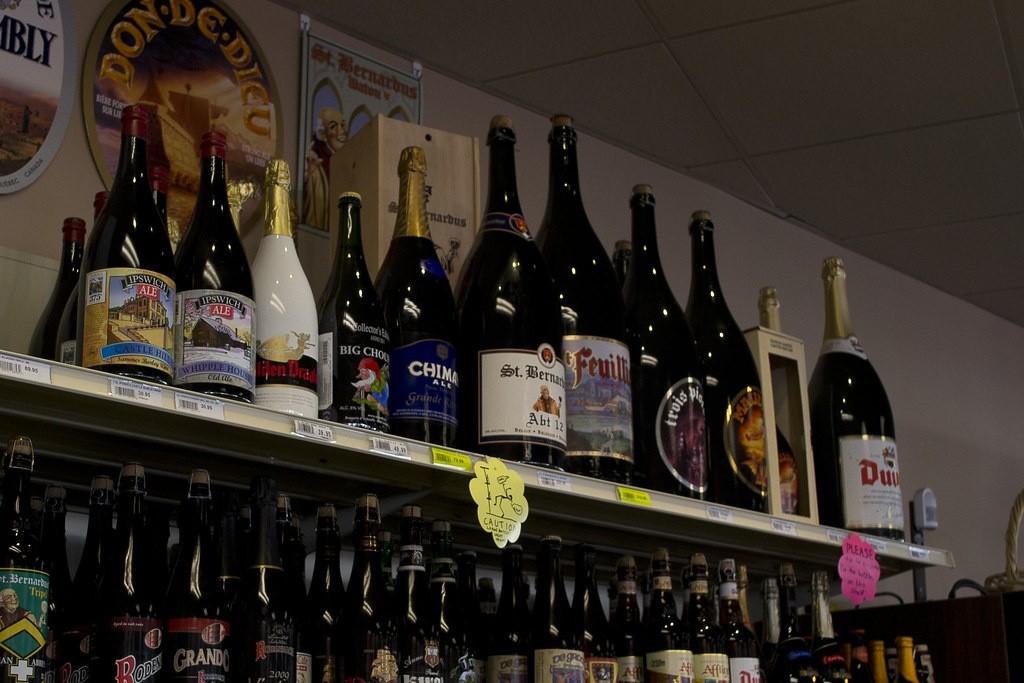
[374,146,458,449]
[226,477,311,683]
[534,115,634,487]
[608,547,693,683]
[454,115,566,472]
[684,210,769,514]
[759,287,781,333]
[622,184,709,501]
[74,104,176,386]
[174,132,256,405]
[497,535,619,683]
[760,564,936,683]
[73,461,228,683]
[28,191,112,366]
[0,436,79,683]
[613,241,633,293]
[316,191,389,434]
[808,257,906,542]
[681,553,760,683]
[251,159,319,420]
[308,494,396,683]
[395,504,497,683]
